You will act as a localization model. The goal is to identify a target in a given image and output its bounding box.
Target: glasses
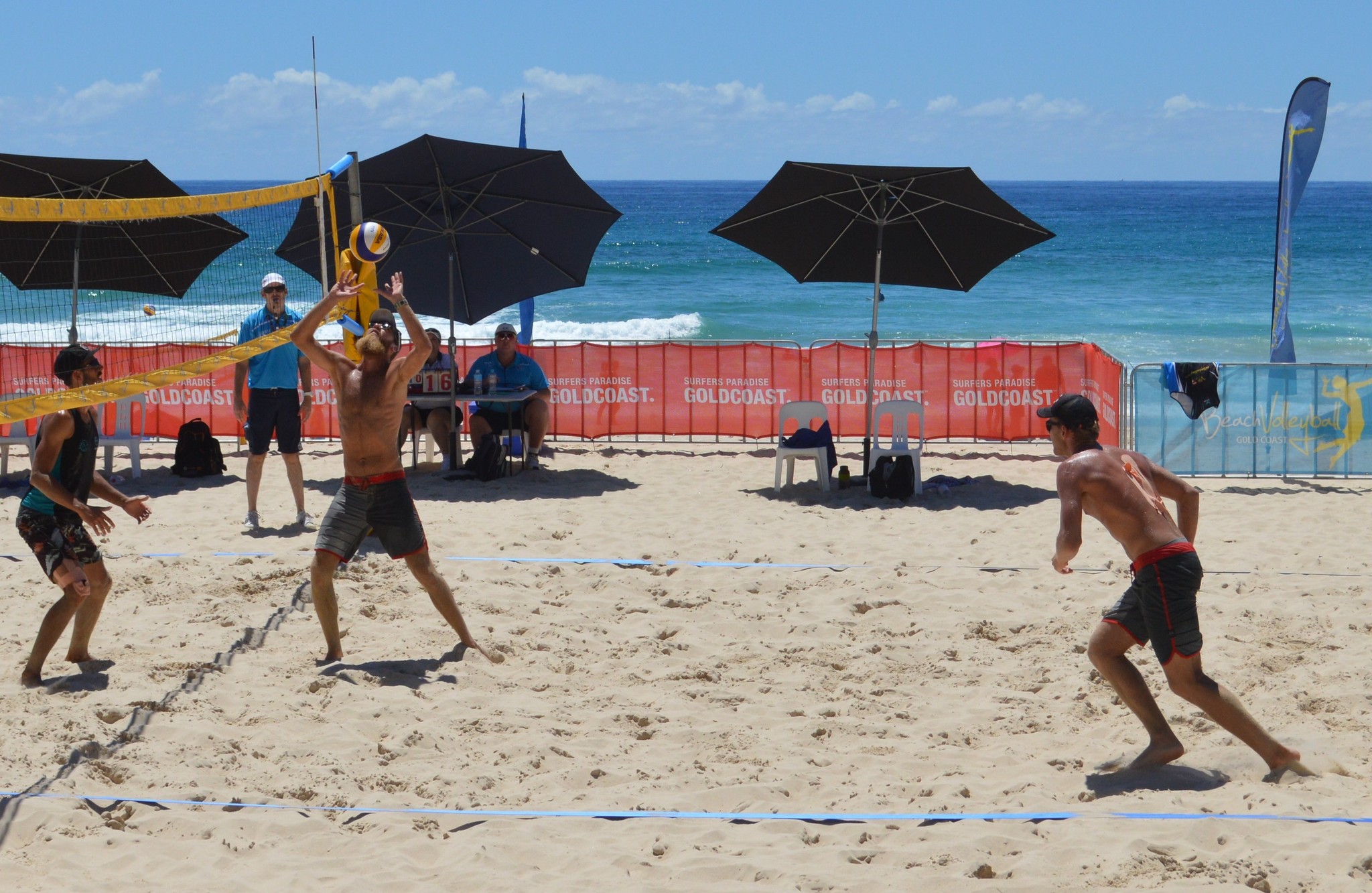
[1046,419,1058,433]
[263,284,285,293]
[496,334,517,340]
[369,322,391,329]
[82,358,100,371]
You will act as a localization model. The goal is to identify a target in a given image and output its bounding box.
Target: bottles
[110,476,125,485]
[936,485,950,494]
[839,466,850,487]
[474,369,482,395]
[488,369,496,393]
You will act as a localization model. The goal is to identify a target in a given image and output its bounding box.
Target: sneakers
[244,509,261,529]
[296,508,316,530]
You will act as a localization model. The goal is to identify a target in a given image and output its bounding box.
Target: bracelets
[392,298,409,309]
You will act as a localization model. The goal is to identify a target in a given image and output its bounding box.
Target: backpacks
[171,418,227,478]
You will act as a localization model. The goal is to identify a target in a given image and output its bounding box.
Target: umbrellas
[0,152,250,344]
[274,134,624,471]
[707,160,1057,478]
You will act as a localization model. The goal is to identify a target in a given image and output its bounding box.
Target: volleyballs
[349,222,391,262]
[144,304,155,316]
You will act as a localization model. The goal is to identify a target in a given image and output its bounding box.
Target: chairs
[95,392,146,482]
[0,393,41,475]
[866,400,923,495]
[774,401,831,493]
[412,425,463,467]
[500,428,530,462]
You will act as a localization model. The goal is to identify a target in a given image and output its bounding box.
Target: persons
[397,328,464,469]
[1036,392,1301,775]
[233,273,316,531]
[465,323,552,470]
[291,269,491,660]
[16,344,151,684]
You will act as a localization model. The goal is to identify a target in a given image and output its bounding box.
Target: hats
[425,328,441,341]
[262,273,285,287]
[1036,394,1098,420]
[369,309,401,345]
[495,323,517,335]
[54,345,100,381]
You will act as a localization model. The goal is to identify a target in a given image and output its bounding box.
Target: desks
[398,389,537,479]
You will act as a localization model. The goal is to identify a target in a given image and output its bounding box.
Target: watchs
[303,391,314,398]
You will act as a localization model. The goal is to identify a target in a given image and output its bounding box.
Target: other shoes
[525,454,541,470]
[441,457,450,470]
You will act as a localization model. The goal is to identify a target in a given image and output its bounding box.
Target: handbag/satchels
[465,434,508,481]
[868,455,915,502]
